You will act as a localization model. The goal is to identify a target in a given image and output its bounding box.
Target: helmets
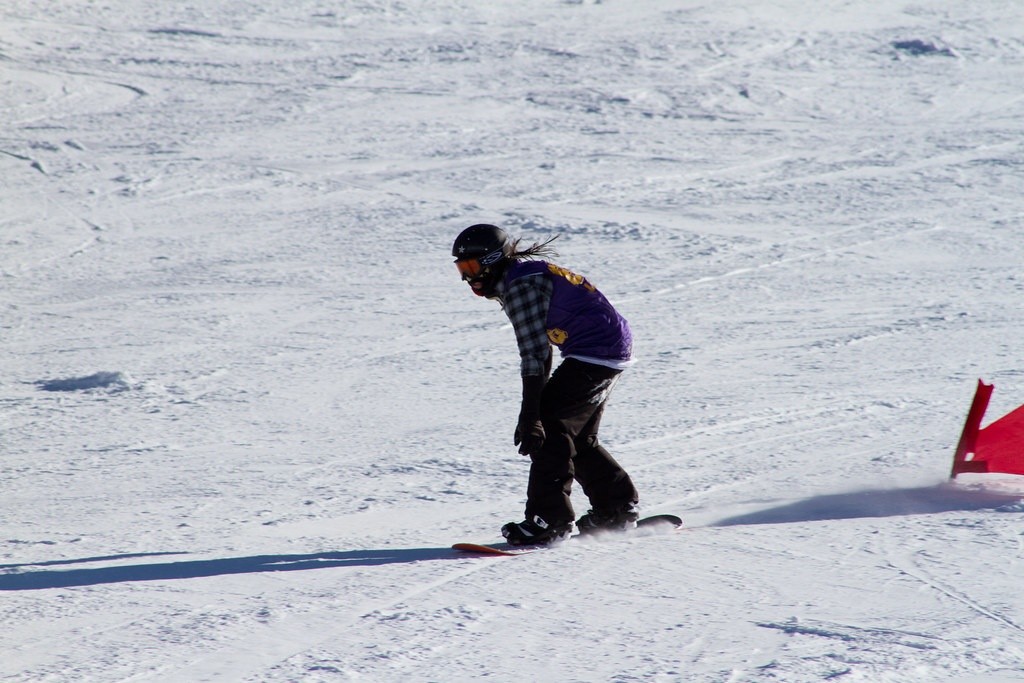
[452,224,512,280]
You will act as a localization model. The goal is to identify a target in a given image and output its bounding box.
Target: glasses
[455,257,483,280]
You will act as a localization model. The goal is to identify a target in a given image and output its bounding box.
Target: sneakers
[578,500,639,535]
[500,512,573,544]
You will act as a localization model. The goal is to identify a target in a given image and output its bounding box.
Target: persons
[451,223,639,548]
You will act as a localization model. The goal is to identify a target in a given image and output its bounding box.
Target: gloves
[514,399,546,456]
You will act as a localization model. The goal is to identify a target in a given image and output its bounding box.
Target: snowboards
[452,513,683,555]
